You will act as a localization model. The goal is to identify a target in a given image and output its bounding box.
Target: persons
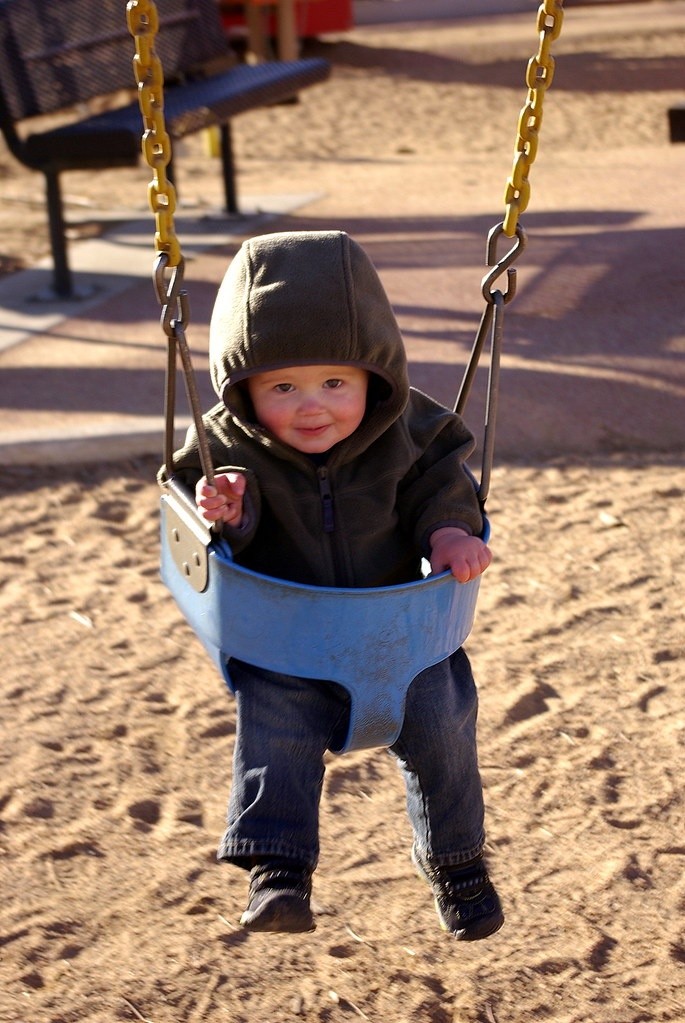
[175,230,505,941]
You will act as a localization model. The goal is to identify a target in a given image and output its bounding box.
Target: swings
[120,0,567,754]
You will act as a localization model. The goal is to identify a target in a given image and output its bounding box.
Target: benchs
[0,0,333,306]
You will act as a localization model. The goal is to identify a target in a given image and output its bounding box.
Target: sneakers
[239,864,313,933]
[410,841,505,942]
